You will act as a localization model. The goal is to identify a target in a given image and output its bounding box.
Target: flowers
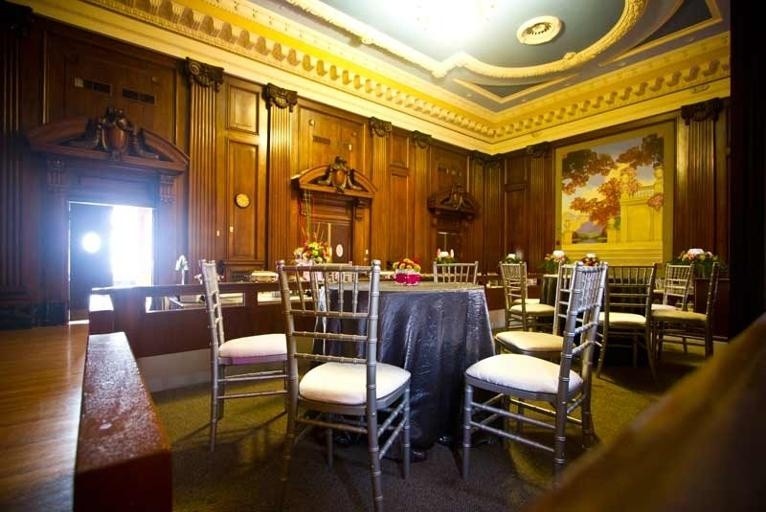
[676,248,730,274]
[435,247,456,263]
[498,253,524,265]
[575,253,601,266]
[293,219,333,264]
[538,250,571,270]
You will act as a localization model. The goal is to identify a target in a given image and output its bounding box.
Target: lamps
[515,14,562,45]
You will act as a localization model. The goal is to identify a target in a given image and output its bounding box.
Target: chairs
[275,258,414,509]
[493,262,576,430]
[460,260,613,509]
[651,260,720,375]
[591,263,660,379]
[499,259,556,335]
[199,258,298,455]
[327,259,354,283]
[433,260,480,284]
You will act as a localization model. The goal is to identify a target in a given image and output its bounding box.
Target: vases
[302,265,324,281]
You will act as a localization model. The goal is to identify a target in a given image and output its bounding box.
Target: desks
[538,272,649,336]
[310,279,506,462]
